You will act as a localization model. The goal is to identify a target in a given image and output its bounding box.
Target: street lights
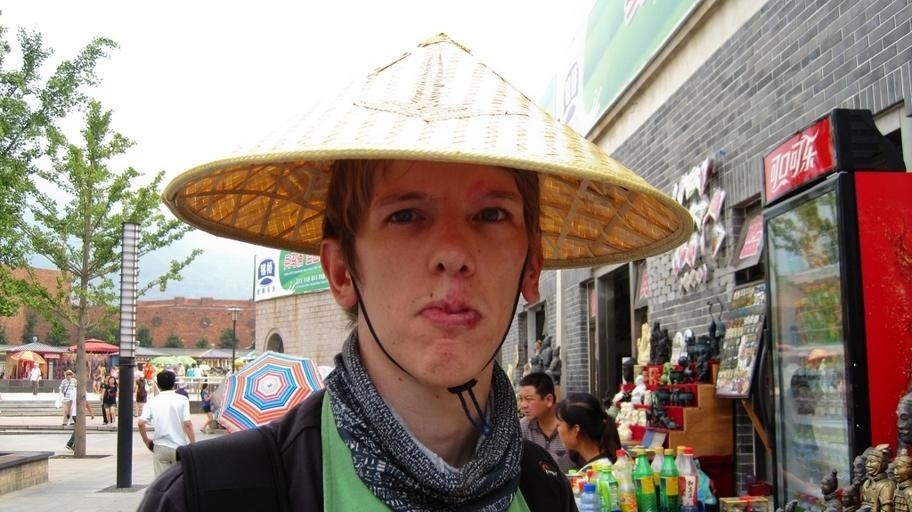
[114,219,143,486]
[225,307,242,374]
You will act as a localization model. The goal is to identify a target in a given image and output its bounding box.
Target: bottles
[563,446,698,512]
[771,196,850,512]
[642,370,649,384]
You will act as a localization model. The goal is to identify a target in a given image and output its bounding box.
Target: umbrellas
[216,350,325,437]
[11,352,45,364]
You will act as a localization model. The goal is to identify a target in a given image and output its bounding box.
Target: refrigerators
[756,107,911,508]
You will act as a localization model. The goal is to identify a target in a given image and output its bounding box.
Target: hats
[160,31,694,270]
[63,369,75,375]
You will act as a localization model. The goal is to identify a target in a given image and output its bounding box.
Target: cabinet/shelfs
[614,360,736,512]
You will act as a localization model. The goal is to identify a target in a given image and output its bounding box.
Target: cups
[633,364,644,384]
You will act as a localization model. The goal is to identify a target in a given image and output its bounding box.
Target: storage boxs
[719,495,769,512]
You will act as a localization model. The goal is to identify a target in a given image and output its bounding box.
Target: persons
[517,370,578,472]
[554,393,621,474]
[819,392,912,512]
[60,355,245,480]
[31,362,41,395]
[530,335,560,385]
[136,33,693,512]
